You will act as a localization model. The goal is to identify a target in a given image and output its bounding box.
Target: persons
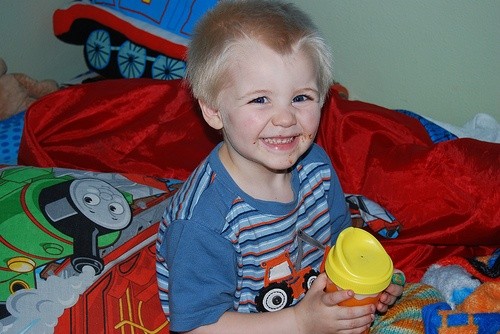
[155,0,405,334]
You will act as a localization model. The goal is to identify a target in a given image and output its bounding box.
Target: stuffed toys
[0,58,58,120]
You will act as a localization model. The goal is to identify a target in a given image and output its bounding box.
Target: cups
[323,227,393,334]
